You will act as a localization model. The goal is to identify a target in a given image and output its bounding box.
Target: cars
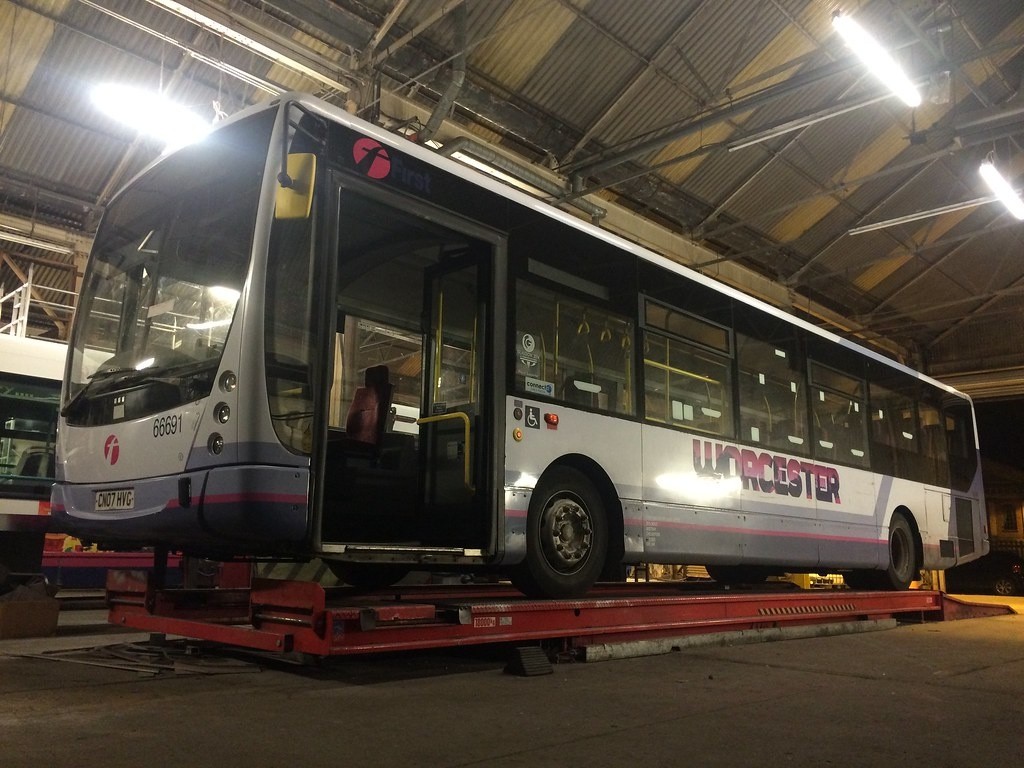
[949,553,1024,596]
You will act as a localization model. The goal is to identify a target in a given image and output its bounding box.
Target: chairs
[559,372,590,406]
[697,413,947,462]
[326,364,391,458]
[13,446,56,486]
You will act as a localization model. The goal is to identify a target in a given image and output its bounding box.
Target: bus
[50,89,987,595]
[0,333,132,599]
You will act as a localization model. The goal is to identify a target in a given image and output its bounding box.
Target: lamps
[978,139,1024,220]
[831,0,922,108]
